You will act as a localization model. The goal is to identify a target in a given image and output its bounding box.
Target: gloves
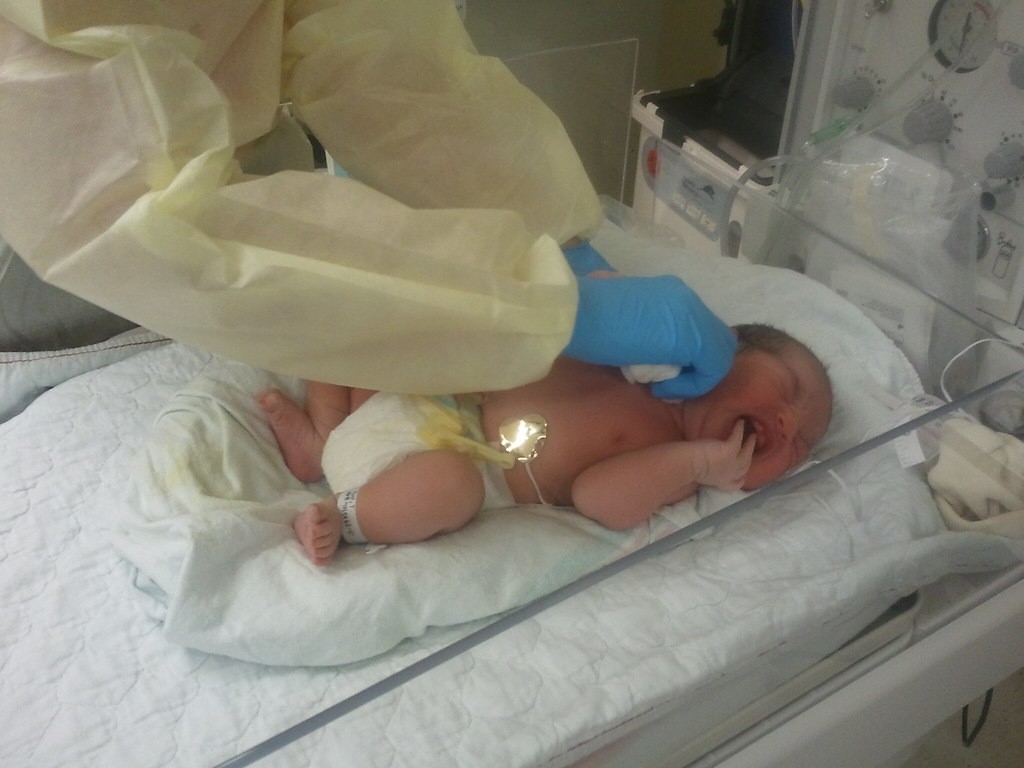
[560,239,739,400]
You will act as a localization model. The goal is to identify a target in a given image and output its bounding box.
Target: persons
[0,0,738,401]
[254,324,833,564]
